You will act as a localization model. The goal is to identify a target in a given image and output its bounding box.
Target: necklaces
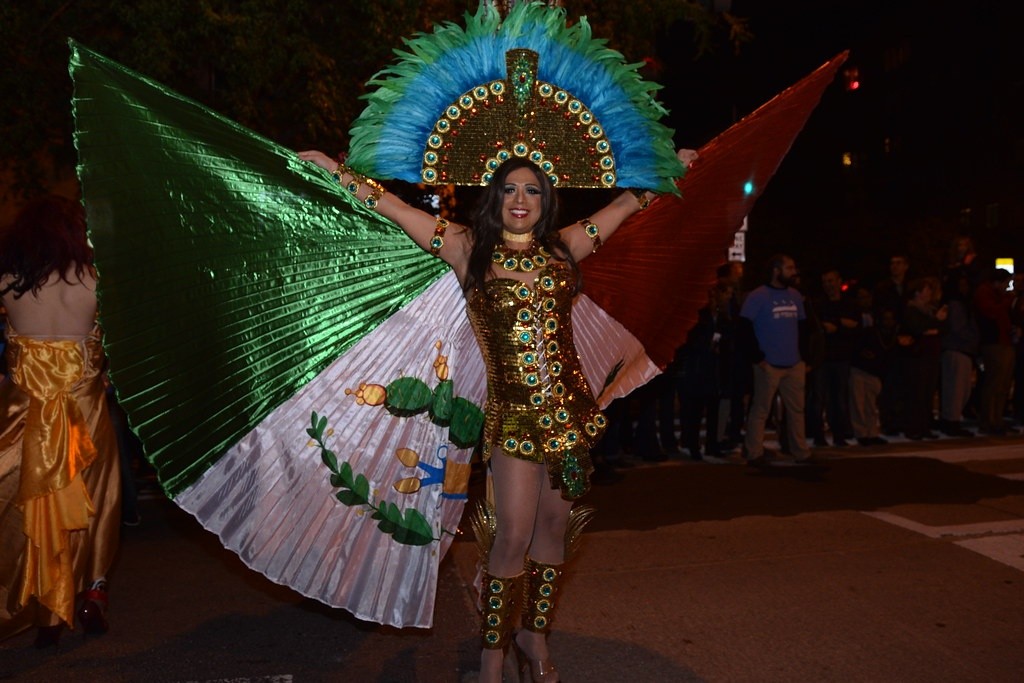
[490,239,552,273]
[501,229,533,244]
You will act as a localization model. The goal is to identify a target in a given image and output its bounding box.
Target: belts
[766,361,799,369]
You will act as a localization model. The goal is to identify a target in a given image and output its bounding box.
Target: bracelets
[627,187,650,209]
[332,162,386,209]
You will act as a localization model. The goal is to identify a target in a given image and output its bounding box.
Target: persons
[592,231,1024,484]
[295,145,701,683]
[0,193,120,641]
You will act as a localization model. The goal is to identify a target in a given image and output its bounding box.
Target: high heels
[511,632,561,683]
[79,588,110,639]
[26,623,68,652]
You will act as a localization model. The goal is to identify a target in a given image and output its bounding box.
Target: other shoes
[813,437,830,447]
[793,454,828,464]
[905,432,923,440]
[947,424,974,437]
[923,429,941,439]
[680,447,702,463]
[977,424,1020,439]
[744,464,763,474]
[586,461,628,483]
[836,437,850,449]
[705,446,728,457]
[858,436,888,447]
